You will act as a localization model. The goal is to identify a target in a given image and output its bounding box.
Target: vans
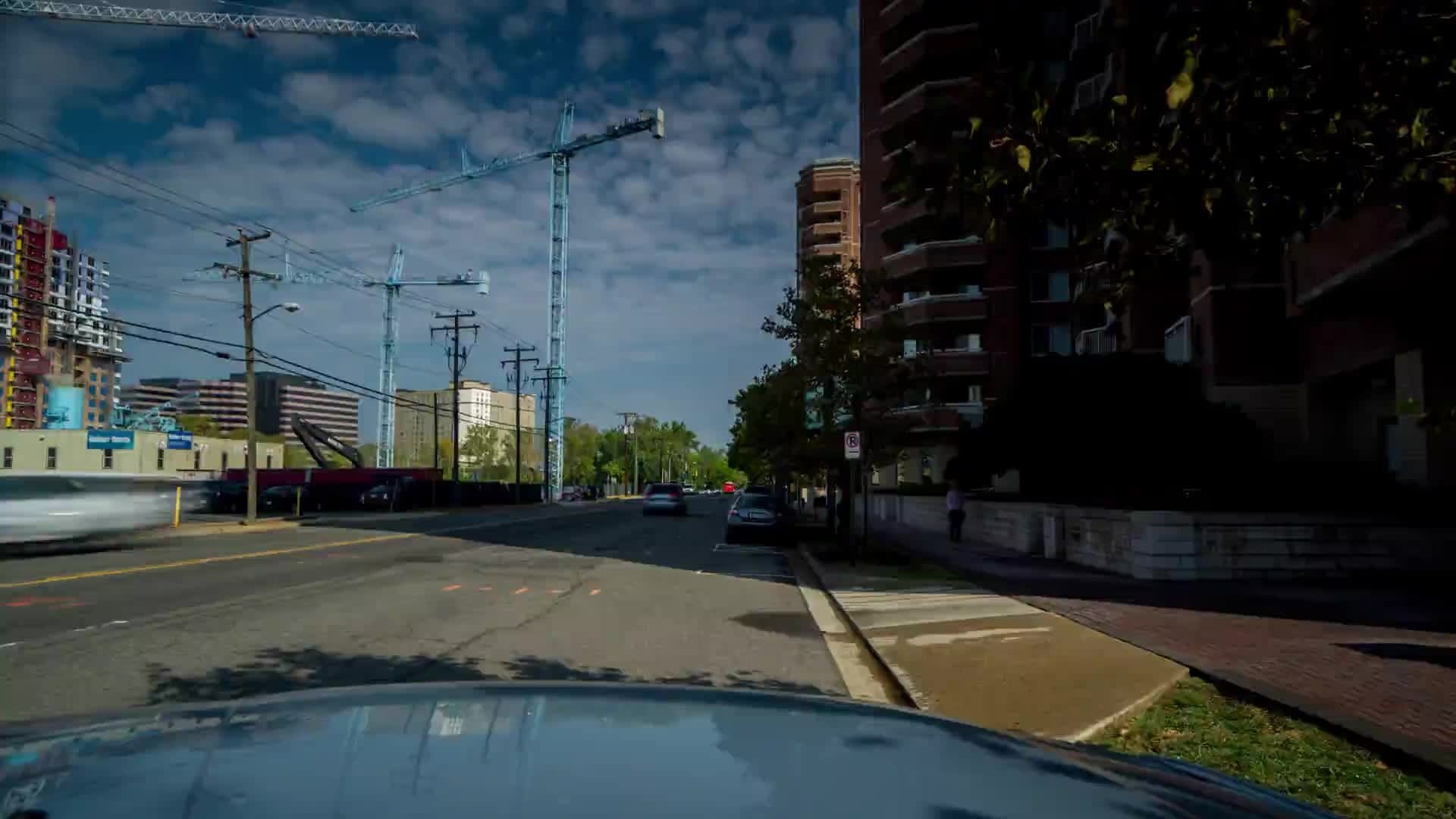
[736,482,773,500]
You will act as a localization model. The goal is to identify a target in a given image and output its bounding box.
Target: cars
[727,491,783,543]
[641,481,686,518]
[190,481,260,514]
[258,484,311,517]
[357,484,400,511]
[683,484,695,495]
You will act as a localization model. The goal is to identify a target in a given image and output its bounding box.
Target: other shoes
[948,538,954,542]
[955,538,961,542]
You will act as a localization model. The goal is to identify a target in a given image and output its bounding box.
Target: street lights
[246,302,300,523]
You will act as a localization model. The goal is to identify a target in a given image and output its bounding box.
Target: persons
[945,479,967,543]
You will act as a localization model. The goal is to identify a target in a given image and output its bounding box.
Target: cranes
[1,1,422,43]
[345,99,667,501]
[179,242,492,469]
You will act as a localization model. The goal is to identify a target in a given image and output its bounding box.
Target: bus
[724,481,735,495]
